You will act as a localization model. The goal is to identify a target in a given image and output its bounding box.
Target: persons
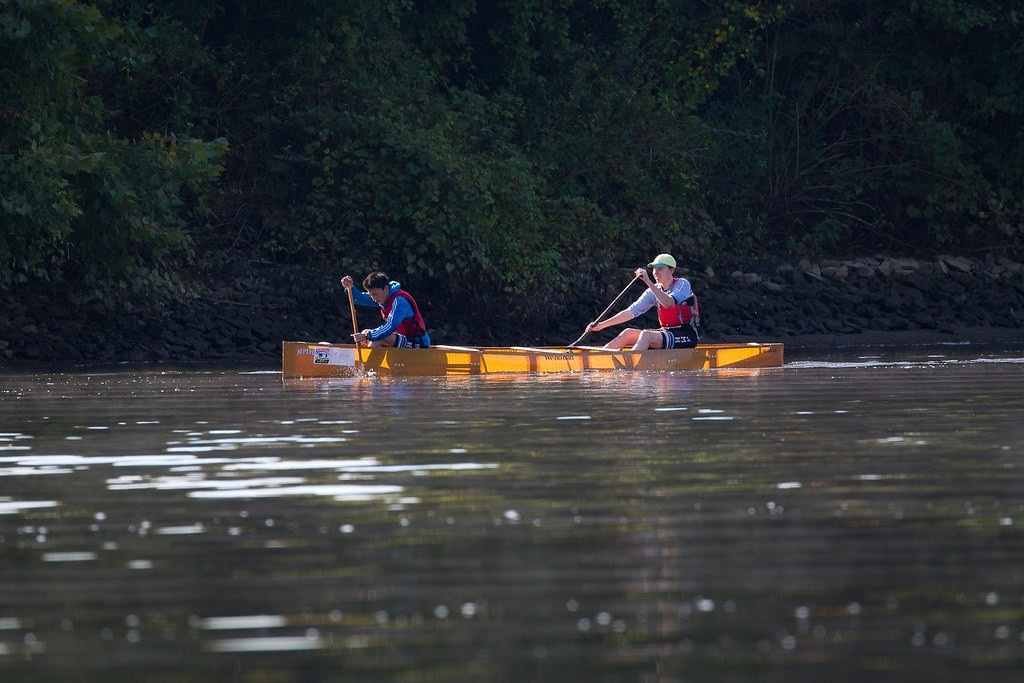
[585,254,700,350]
[341,272,430,349]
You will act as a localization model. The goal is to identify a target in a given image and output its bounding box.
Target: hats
[647,254,676,269]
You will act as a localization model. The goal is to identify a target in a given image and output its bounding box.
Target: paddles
[570,273,644,347]
[345,282,372,372]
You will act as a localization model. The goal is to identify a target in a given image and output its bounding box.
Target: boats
[282,339,784,380]
